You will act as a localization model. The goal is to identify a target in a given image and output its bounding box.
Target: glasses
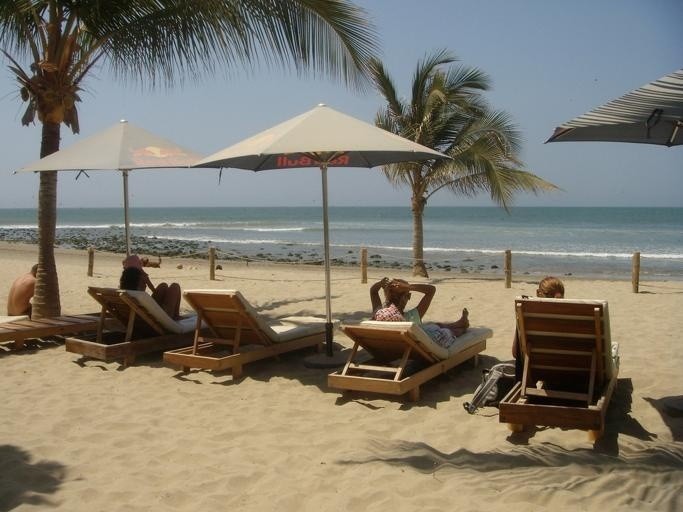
[403,292,411,300]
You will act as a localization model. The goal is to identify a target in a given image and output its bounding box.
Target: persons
[6,265,38,315]
[119,254,182,318]
[368,277,470,347]
[536,276,564,301]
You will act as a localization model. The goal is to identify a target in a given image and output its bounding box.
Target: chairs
[327,317,493,401]
[166,289,337,379]
[66,286,208,368]
[497,295,637,432]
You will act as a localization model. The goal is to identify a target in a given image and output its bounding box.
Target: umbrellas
[14,120,194,254]
[194,103,454,369]
[543,69,683,147]
